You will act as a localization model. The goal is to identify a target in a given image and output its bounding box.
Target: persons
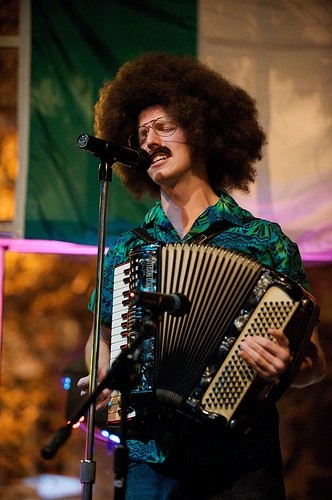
[76,53,326,500]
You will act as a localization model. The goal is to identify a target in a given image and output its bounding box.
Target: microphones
[130,291,190,317]
[76,134,151,174]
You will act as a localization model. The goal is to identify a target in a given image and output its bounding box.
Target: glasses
[128,116,181,149]
